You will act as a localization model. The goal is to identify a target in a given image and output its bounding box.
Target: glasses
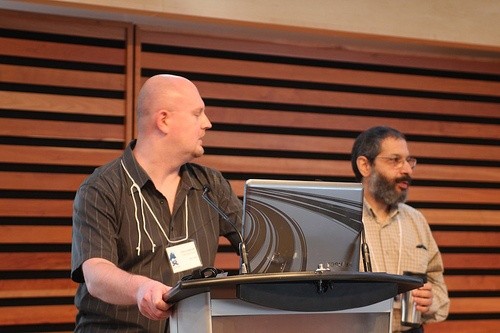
[367,154,416,169]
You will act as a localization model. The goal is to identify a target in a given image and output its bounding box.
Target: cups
[400,270,429,328]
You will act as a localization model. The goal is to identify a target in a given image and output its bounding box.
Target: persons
[351,126,449,333]
[70,74,244,333]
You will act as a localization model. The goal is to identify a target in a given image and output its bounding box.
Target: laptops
[239,179,365,276]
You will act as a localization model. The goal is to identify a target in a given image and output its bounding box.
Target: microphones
[200,183,250,275]
